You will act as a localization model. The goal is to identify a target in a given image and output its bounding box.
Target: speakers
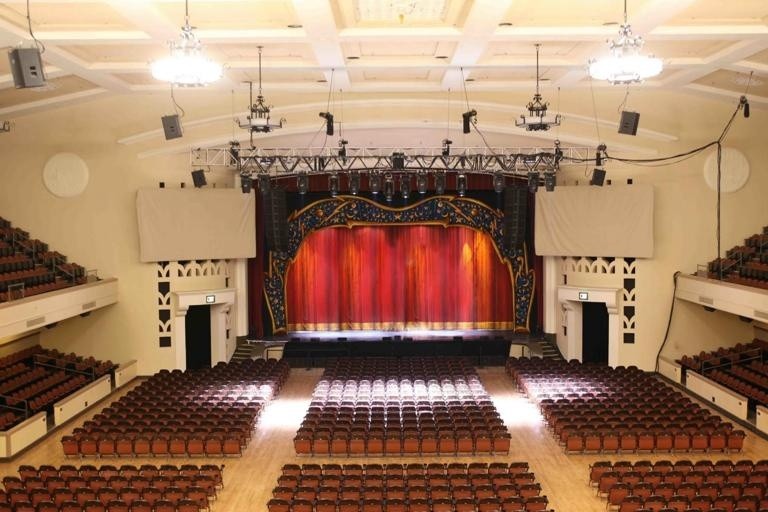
[591,169,605,186]
[162,115,182,140]
[618,111,639,135]
[9,49,47,89]
[192,170,206,186]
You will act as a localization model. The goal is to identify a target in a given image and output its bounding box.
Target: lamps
[588,0,663,83]
[516,42,560,131]
[234,44,284,131]
[149,2,222,88]
[241,153,561,200]
